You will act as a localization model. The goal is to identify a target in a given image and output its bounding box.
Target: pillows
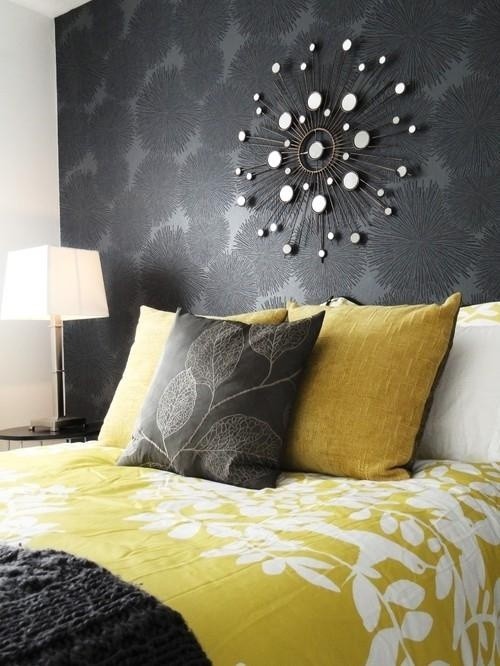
[115,310,326,489]
[411,300,500,463]
[269,293,462,481]
[99,305,288,447]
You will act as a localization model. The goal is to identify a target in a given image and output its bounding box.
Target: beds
[0,440,500,666]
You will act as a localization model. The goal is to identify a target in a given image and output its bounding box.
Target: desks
[0,426,100,451]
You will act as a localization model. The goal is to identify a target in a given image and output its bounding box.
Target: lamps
[0,245,111,433]
